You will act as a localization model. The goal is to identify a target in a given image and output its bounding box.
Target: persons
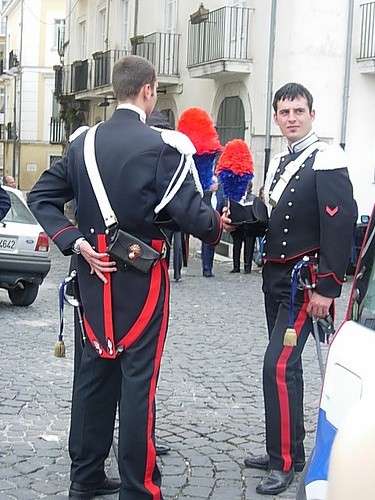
[222,81,357,494]
[0,56,264,500]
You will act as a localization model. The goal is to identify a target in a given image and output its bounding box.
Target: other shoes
[230,268,240,273]
[203,270,215,277]
[245,266,251,274]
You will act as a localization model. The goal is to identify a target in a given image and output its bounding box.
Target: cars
[0,185,51,307]
[296,193,375,500]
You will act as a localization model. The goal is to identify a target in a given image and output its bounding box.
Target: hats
[226,197,268,239]
[152,191,214,233]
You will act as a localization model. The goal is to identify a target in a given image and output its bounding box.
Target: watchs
[71,237,86,255]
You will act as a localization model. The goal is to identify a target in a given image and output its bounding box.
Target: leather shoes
[69,471,120,500]
[244,454,306,472]
[256,466,295,495]
[155,444,170,455]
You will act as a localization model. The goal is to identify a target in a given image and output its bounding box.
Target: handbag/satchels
[108,229,160,276]
